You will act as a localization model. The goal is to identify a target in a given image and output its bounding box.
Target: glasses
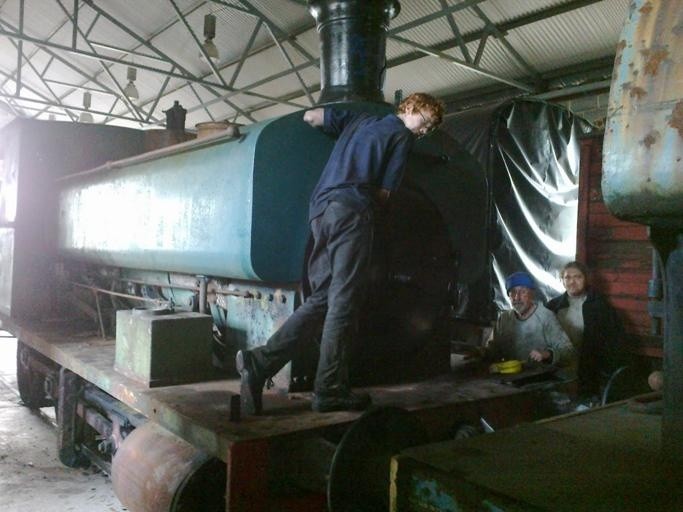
[415,108,438,132]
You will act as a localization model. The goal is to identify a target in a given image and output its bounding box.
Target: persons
[475,262,613,383]
[236,92,445,415]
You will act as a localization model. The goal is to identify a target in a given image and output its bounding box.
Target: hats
[505,272,535,297]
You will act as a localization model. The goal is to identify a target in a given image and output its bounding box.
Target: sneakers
[312,391,370,413]
[235,350,265,414]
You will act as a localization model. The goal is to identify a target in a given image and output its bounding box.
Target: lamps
[80,91,93,124]
[199,8,222,62]
[125,67,143,101]
